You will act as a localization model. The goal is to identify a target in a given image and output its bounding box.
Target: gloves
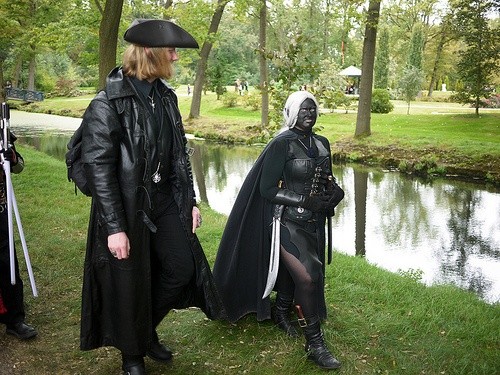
[0,148,18,165]
[307,190,338,215]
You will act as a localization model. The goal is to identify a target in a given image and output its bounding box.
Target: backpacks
[65,87,109,197]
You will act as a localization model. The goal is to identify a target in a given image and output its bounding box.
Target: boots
[297,315,342,368]
[270,293,303,338]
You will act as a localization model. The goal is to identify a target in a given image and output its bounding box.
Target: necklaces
[298,135,312,151]
[146,89,156,113]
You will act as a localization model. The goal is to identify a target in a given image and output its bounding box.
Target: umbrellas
[336,65,361,76]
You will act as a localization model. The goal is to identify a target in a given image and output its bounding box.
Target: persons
[235,78,249,95]
[78,19,211,375]
[346,81,356,94]
[0,120,37,340]
[211,90,345,370]
[299,85,307,91]
[187,84,191,95]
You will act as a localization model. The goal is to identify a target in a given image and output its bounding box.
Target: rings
[112,251,117,256]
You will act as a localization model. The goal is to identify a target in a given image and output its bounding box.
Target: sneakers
[6,319,38,340]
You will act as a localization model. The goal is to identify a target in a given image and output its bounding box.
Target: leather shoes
[144,339,173,362]
[121,355,147,375]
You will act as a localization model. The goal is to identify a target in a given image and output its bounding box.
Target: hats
[123,17,200,48]
